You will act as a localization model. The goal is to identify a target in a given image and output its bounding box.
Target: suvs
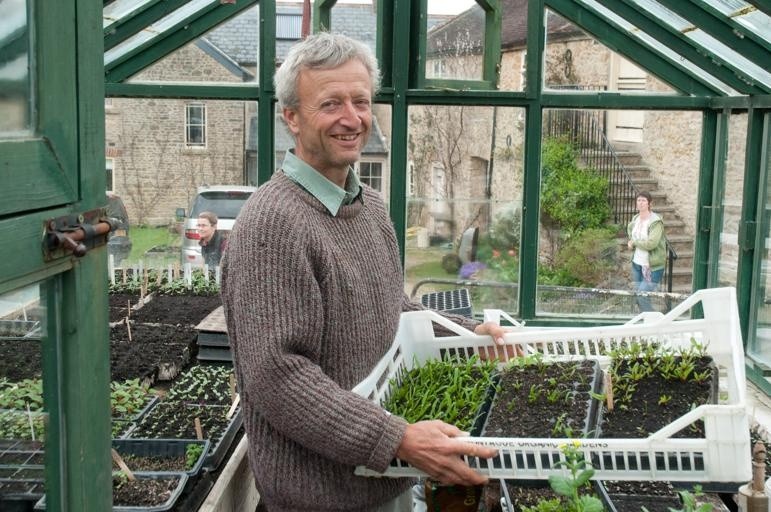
[104,191,132,265]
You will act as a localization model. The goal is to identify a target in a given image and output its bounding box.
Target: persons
[198,212,230,272]
[628,191,669,315]
[217,33,545,511]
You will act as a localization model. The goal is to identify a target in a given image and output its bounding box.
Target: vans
[174,182,260,275]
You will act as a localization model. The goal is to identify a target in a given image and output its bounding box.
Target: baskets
[349,286,754,485]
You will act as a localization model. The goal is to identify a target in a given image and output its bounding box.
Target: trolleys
[441,206,485,274]
